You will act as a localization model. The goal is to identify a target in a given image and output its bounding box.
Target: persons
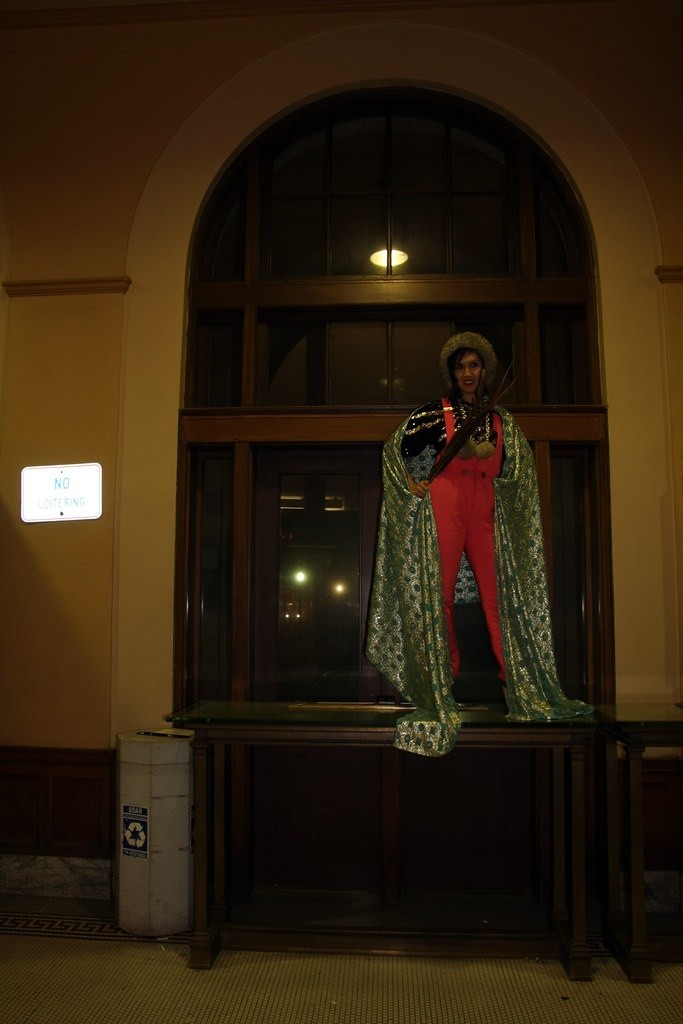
[365,333,594,758]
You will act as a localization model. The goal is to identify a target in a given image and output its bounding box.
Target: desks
[164,700,615,981]
[595,705,683,984]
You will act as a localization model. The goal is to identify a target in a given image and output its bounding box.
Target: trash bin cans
[115,727,195,936]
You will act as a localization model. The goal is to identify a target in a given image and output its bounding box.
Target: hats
[439,331,496,393]
